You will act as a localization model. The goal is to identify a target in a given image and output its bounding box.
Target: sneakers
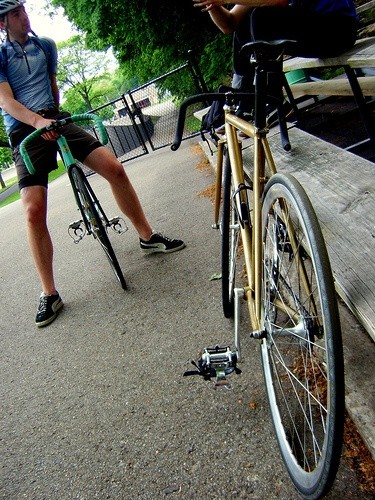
[238,99,295,137]
[217,105,255,134]
[139,232,184,254]
[36,291,64,328]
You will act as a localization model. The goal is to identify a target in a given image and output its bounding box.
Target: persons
[0,0,185,326]
[192,0,358,124]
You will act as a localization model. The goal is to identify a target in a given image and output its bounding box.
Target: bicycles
[171,39,346,500]
[20,113,129,291]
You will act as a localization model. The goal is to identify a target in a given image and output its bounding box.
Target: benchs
[193,0,375,342]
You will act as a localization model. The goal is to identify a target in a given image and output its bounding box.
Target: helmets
[0,0,25,15]
